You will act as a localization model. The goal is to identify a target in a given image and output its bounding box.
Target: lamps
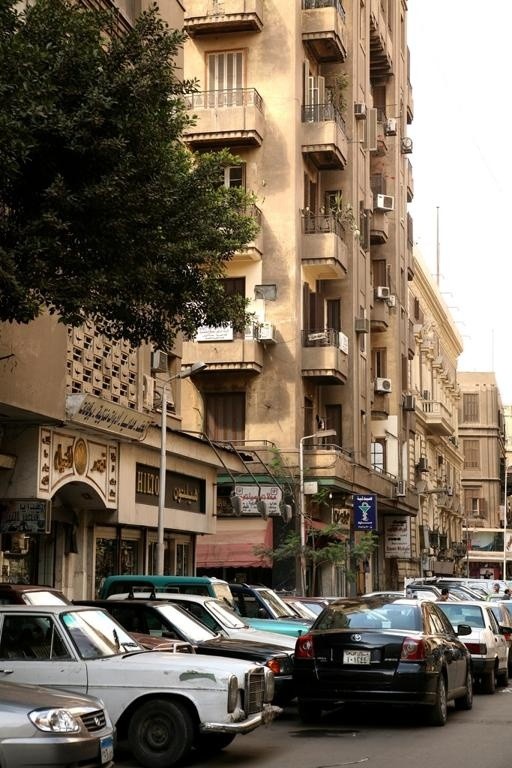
[181,430,293,523]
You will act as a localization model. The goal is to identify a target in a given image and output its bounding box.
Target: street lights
[155,361,207,575]
[299,428,336,596]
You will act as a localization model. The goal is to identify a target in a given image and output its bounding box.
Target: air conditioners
[258,323,276,340]
[419,458,428,469]
[405,396,416,408]
[386,118,396,132]
[354,103,368,120]
[356,318,370,332]
[373,193,395,211]
[375,377,392,393]
[142,350,168,411]
[375,286,396,308]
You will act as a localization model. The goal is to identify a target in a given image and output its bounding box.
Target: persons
[491,583,501,594]
[501,589,512,600]
[434,588,450,602]
[478,569,493,579]
